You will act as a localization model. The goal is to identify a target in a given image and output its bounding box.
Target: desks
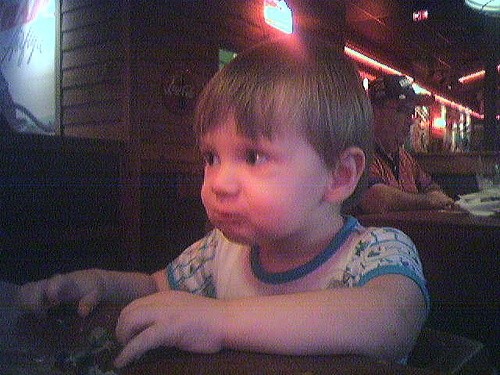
[354,211,500,372]
[0,297,489,375]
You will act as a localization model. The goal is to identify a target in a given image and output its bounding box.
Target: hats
[369,74,436,109]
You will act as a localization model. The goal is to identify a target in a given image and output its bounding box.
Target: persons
[342,74,454,214]
[13,31,430,367]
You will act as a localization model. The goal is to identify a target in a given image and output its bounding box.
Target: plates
[454,202,500,212]
